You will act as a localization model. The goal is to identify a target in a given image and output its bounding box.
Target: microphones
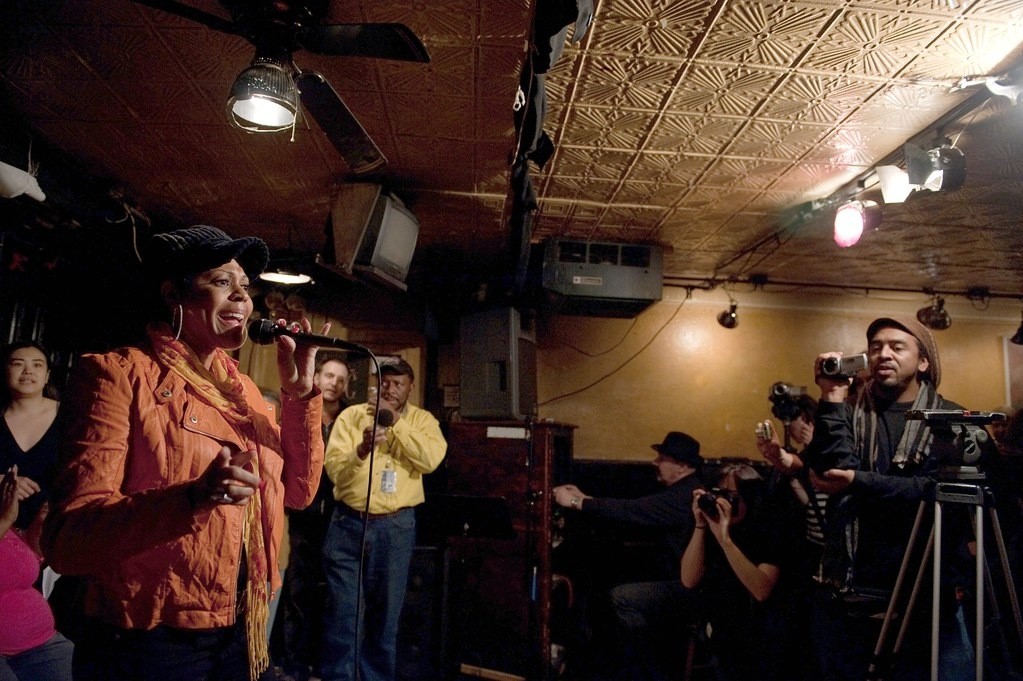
[248,318,370,353]
[378,409,394,430]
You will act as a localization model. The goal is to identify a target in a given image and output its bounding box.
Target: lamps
[832,200,882,248]
[917,292,952,330]
[876,124,966,204]
[716,301,739,328]
[222,49,302,132]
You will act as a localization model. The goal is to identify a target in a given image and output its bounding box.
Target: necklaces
[384,428,395,465]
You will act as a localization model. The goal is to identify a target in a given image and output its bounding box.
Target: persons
[322,357,447,681]
[38,226,333,681]
[551,318,1023,681]
[0,465,74,681]
[0,342,76,643]
[283,357,349,681]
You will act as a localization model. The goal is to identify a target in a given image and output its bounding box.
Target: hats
[142,226,269,313]
[375,357,415,381]
[650,432,704,465]
[867,316,940,389]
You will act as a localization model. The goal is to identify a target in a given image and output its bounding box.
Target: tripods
[868,466,1023,681]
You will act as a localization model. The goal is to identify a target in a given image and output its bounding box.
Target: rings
[221,489,227,499]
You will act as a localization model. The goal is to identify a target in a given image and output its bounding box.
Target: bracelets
[695,526,705,529]
[571,497,577,509]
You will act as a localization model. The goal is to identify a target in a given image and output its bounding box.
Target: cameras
[697,488,739,517]
[772,383,807,400]
[754,423,772,442]
[823,353,868,377]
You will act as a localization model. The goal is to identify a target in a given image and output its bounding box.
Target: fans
[133,0,429,176]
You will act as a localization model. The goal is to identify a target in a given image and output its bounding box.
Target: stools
[681,620,721,680]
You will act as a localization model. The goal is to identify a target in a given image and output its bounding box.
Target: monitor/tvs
[353,193,420,283]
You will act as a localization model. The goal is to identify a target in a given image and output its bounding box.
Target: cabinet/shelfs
[442,420,579,679]
